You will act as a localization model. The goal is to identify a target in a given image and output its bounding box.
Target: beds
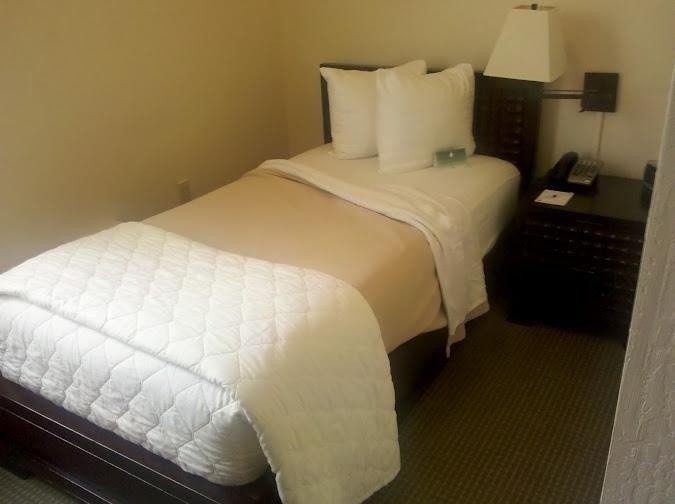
[0,63,545,504]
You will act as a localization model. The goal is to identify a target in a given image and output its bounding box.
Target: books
[535,189,575,208]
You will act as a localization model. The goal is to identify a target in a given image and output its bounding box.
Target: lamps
[482,4,620,114]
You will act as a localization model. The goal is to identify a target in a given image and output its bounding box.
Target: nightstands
[505,176,651,340]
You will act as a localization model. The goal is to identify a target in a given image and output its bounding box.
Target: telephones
[544,151,599,192]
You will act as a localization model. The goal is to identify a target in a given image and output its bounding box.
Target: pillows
[319,59,478,174]
